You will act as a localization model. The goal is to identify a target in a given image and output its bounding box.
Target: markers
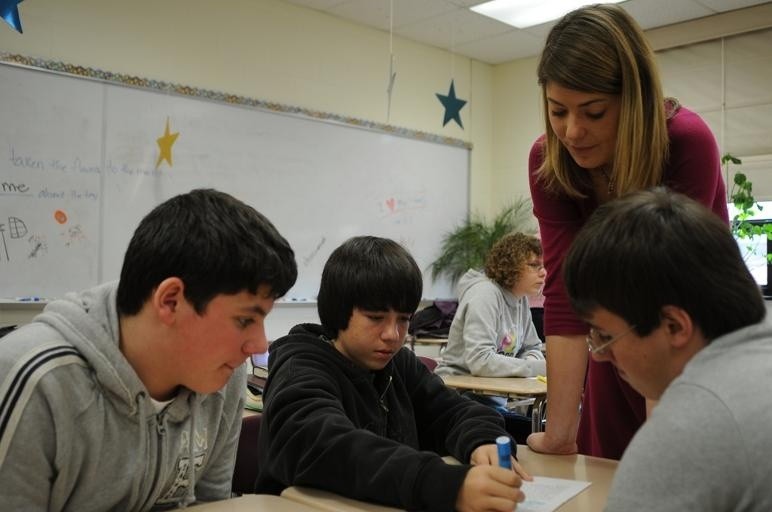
[19,298,39,301]
[292,298,307,301]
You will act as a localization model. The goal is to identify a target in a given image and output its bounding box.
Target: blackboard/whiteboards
[0,53,472,312]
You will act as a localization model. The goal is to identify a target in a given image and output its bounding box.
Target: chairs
[230,415,262,494]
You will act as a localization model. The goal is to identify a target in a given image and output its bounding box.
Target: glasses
[584,324,637,357]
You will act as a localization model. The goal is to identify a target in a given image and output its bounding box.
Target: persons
[527,5,730,460]
[561,186,772,512]
[0,189,298,512]
[253,236,533,512]
[433,232,548,444]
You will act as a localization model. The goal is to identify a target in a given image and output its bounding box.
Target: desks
[405,335,548,436]
[280,443,624,512]
[170,493,332,512]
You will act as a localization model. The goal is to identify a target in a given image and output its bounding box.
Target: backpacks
[408,301,458,350]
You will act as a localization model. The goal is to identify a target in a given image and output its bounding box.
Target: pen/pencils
[497,436,512,471]
[538,375,547,383]
